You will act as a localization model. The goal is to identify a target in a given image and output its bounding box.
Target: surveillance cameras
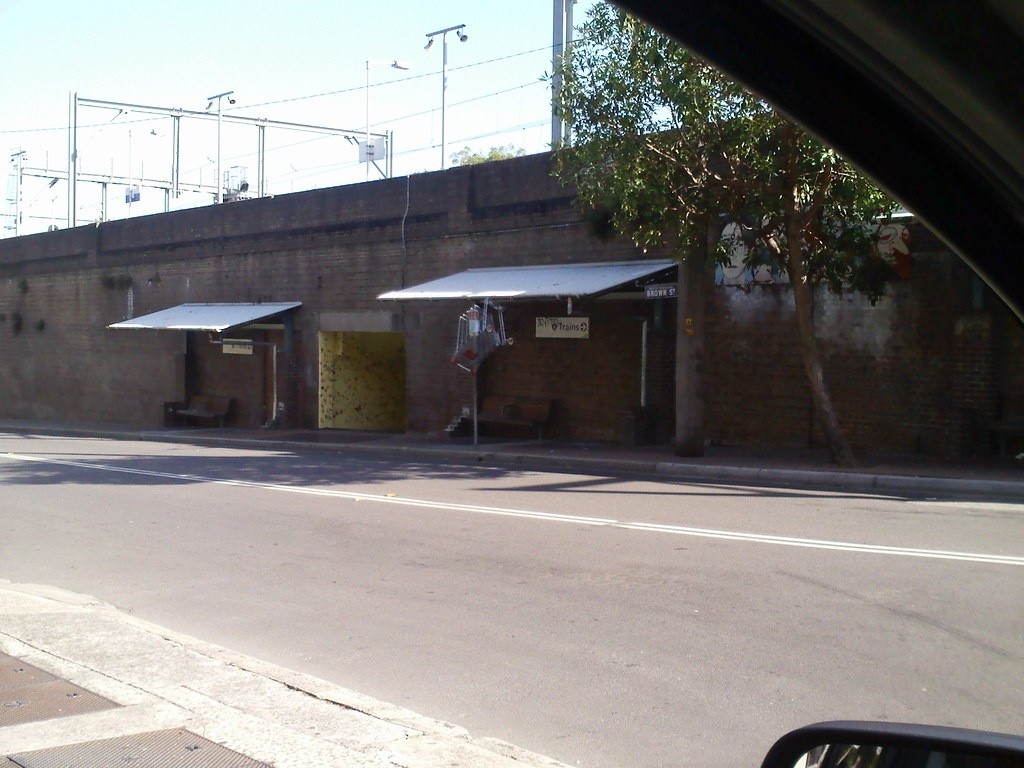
[228,95,236,105]
[240,180,249,193]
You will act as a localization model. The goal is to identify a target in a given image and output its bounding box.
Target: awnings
[378,252,679,306]
[104,299,304,333]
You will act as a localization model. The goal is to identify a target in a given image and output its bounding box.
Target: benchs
[176,395,229,428]
[476,393,556,441]
